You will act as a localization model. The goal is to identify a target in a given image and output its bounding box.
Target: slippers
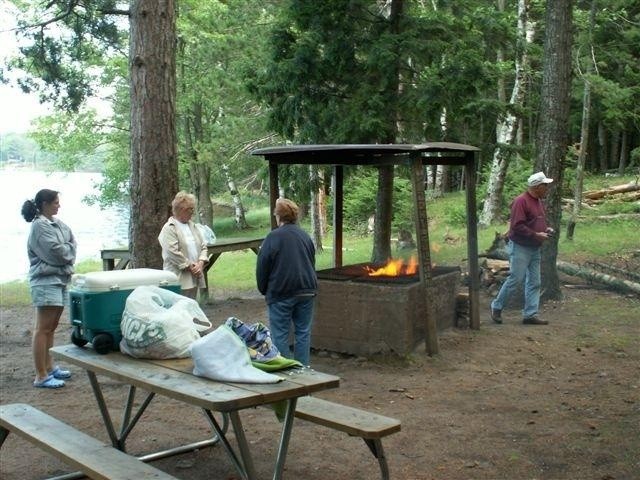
[33,374,64,388]
[49,367,71,379]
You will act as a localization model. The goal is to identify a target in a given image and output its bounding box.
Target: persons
[489,170,555,325]
[157,190,208,303]
[20,188,77,388]
[255,196,318,367]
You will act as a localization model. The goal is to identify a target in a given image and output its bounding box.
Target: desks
[50,344,340,479]
[100,237,265,300]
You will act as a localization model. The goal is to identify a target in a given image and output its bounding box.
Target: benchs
[265,397,403,479]
[0,403,179,479]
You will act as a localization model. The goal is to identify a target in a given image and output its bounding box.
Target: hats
[528,172,553,186]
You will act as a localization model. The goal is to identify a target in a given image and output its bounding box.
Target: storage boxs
[69,268,182,354]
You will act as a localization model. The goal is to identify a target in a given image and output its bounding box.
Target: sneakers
[523,316,547,324]
[492,308,503,324]
